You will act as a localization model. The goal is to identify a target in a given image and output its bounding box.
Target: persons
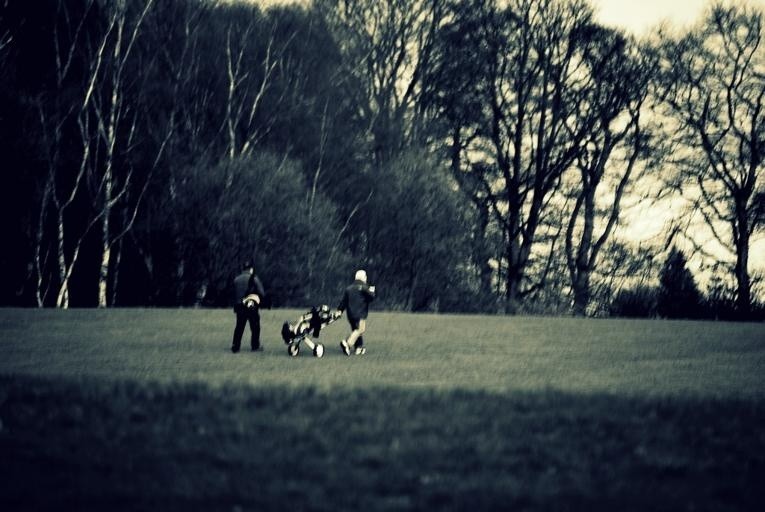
[228,262,267,352]
[333,269,375,356]
[294,304,330,335]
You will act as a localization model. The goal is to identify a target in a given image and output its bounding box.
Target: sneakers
[355,347,366,355]
[340,340,350,356]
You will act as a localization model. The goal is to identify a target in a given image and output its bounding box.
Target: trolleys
[281,305,342,358]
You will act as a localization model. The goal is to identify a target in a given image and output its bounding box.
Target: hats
[355,270,367,284]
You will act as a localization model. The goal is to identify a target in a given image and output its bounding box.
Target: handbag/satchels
[242,294,260,309]
